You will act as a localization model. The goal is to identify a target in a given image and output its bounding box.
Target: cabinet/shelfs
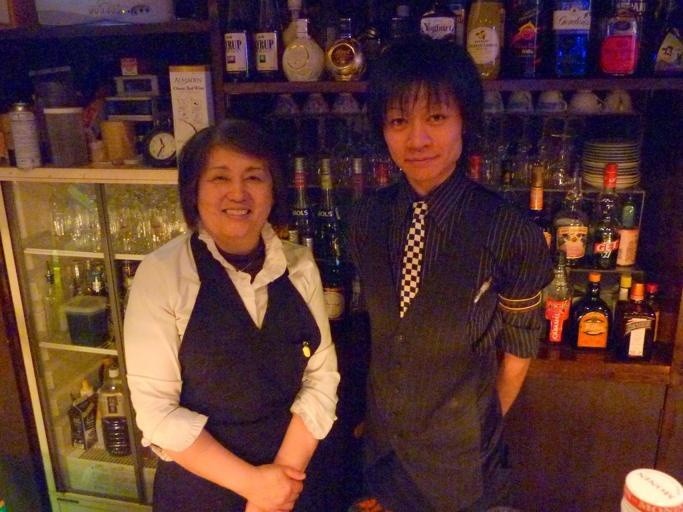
[499,365,682,508]
[210,0,683,386]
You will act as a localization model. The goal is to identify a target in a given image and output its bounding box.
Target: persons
[123,120,342,512]
[325,31,555,511]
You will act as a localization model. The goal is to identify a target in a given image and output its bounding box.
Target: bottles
[41,259,131,346]
[543,253,661,361]
[79,356,130,458]
[416,0,683,79]
[554,162,640,269]
[9,102,39,169]
[289,154,312,249]
[224,0,383,83]
[312,157,348,321]
[497,158,521,208]
[350,150,390,203]
[525,165,551,250]
[619,466,683,512]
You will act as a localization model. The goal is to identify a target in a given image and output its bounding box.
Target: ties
[398,201,429,319]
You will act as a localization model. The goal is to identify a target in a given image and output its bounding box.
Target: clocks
[143,128,176,168]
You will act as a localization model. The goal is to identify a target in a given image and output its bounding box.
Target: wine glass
[475,118,576,187]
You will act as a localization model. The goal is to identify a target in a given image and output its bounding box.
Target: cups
[272,94,366,116]
[42,104,86,167]
[483,91,634,117]
[101,121,136,163]
[43,184,184,252]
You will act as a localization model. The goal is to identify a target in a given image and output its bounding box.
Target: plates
[582,137,640,189]
